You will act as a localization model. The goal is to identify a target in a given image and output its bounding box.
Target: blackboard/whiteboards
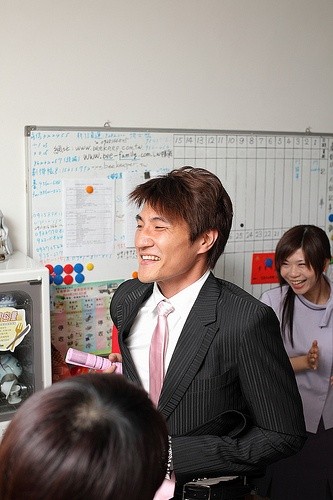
[24,124,333,366]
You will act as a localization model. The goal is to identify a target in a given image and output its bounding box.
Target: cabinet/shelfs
[0,251,53,440]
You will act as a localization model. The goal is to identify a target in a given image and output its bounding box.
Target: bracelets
[164,435,172,480]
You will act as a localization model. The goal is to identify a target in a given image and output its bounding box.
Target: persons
[99,166,306,500]
[0,373,169,500]
[260,225,333,500]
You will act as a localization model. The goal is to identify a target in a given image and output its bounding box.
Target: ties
[149,301,175,410]
